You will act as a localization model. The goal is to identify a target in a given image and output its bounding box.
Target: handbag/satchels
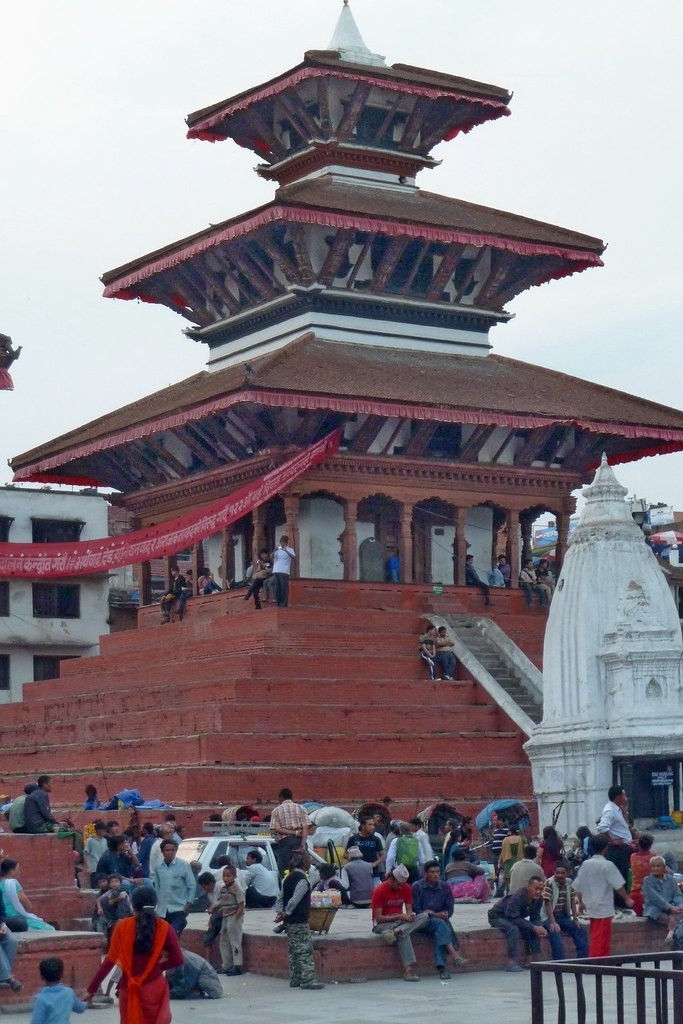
[559,845,571,871]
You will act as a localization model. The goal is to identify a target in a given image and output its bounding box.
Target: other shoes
[435,678,441,681]
[227,966,243,976]
[0,981,11,989]
[404,972,419,981]
[665,938,674,946]
[383,929,397,944]
[160,616,170,624]
[444,675,453,680]
[485,600,496,607]
[212,589,220,595]
[227,579,234,589]
[454,957,467,968]
[494,892,504,897]
[217,968,229,974]
[300,979,325,989]
[505,962,522,972]
[440,971,451,979]
[12,981,23,993]
[175,610,182,621]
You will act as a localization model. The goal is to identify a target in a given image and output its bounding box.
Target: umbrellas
[649,530,683,550]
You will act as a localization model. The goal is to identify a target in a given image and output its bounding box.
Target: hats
[390,820,403,828]
[393,864,409,882]
[349,849,362,860]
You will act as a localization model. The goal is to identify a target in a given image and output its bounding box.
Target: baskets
[308,908,339,931]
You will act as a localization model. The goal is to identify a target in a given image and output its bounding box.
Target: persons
[465,554,555,606]
[0,775,683,1024]
[160,535,296,625]
[419,625,456,681]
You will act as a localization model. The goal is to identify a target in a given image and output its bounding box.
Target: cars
[175,832,329,899]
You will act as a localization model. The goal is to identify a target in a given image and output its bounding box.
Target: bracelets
[296,830,298,836]
